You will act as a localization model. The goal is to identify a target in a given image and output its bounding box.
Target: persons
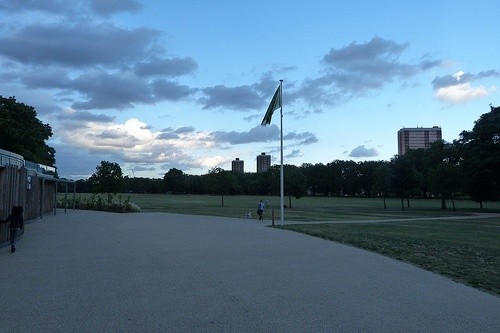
[0,205,23,253]
[257,200,266,221]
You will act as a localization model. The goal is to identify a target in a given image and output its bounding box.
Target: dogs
[245,211,252,219]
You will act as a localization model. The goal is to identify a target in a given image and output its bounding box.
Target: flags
[261,84,281,126]
